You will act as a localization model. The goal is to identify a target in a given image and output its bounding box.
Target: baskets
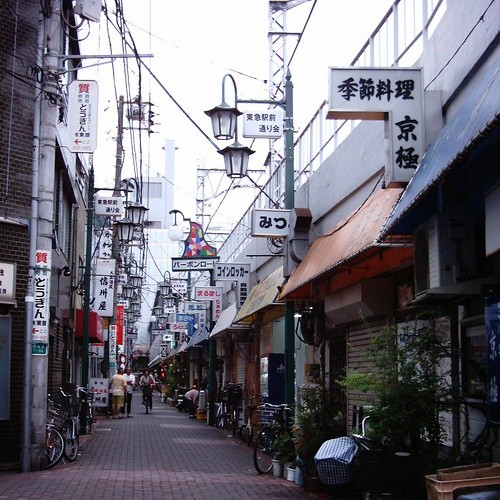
[46,406,67,426]
[259,406,282,424]
[64,396,81,416]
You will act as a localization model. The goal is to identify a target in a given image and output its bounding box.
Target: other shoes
[118,415,123,418]
[127,415,133,417]
[113,416,117,418]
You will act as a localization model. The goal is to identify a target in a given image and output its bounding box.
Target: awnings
[209,302,253,338]
[276,188,413,304]
[148,321,210,367]
[379,58,500,241]
[233,265,288,327]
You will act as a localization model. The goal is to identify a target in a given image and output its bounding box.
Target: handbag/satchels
[314,436,358,483]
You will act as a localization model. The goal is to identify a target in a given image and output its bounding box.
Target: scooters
[177,393,189,412]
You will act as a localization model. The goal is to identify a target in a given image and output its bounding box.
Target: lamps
[58,264,71,278]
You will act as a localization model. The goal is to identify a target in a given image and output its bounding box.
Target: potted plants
[271,383,326,489]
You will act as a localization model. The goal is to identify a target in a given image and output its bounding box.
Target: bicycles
[140,383,154,412]
[211,381,293,475]
[314,412,413,500]
[39,384,95,470]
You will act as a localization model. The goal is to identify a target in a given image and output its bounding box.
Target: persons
[122,367,136,418]
[184,389,199,418]
[109,368,128,418]
[139,370,155,410]
